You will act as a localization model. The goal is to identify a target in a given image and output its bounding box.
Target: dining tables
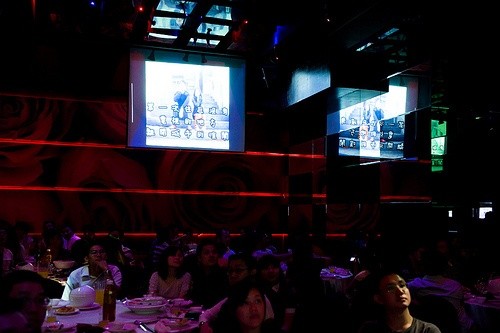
[407,278,500,333]
[45,301,172,333]
[321,268,353,293]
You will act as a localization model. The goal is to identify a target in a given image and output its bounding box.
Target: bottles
[103,269,117,323]
[36,253,49,279]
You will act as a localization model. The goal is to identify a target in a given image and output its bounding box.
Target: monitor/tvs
[128,44,246,152]
[327,79,422,162]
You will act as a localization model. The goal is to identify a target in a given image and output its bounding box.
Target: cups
[93,282,106,305]
[46,305,57,323]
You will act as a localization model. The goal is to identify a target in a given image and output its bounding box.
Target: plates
[79,304,101,309]
[104,321,138,333]
[56,306,79,314]
[159,318,191,327]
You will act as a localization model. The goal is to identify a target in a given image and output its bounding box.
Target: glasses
[228,267,251,275]
[16,292,50,307]
[87,250,105,257]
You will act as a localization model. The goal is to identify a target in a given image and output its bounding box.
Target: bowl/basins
[124,298,168,314]
[70,286,96,307]
[52,259,76,268]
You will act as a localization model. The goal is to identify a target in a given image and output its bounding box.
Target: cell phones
[184,311,198,320]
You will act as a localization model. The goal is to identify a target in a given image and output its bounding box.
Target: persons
[0,219,500,333]
[359,271,442,333]
